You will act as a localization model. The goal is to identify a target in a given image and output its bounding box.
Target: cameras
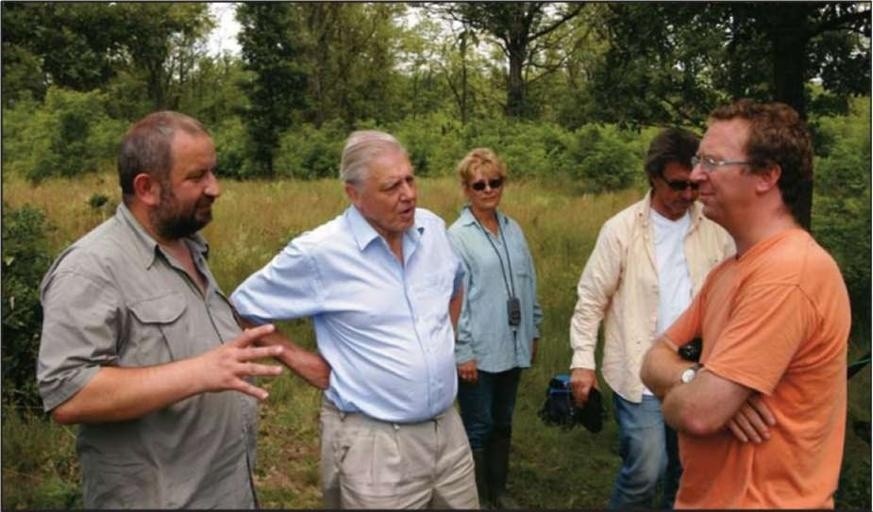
[508,298,523,325]
[539,374,605,432]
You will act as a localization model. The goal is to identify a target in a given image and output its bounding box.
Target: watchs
[680,363,704,383]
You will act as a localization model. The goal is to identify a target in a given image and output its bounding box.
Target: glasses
[689,156,767,171]
[471,179,503,192]
[656,171,699,191]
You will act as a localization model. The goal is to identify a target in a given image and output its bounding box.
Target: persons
[446,143,540,510]
[34,109,284,509]
[227,128,478,510]
[568,123,736,512]
[636,96,854,511]
